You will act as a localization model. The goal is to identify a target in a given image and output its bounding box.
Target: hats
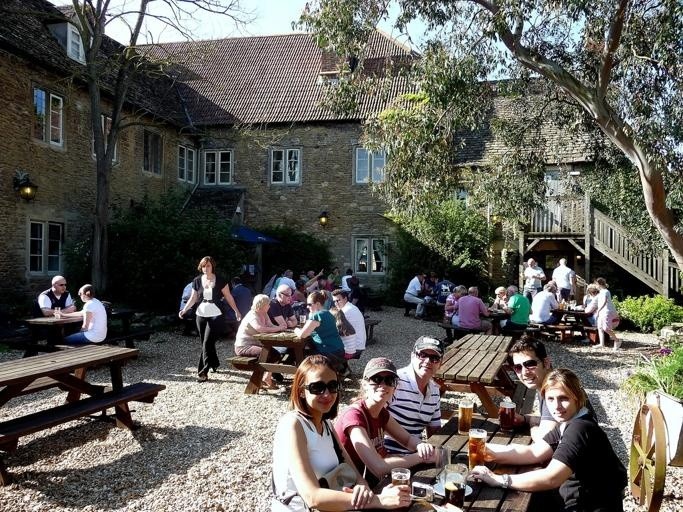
[411,334,444,358]
[362,357,401,380]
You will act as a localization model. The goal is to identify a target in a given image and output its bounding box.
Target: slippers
[261,380,280,390]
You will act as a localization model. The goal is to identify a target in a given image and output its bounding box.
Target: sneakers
[591,343,605,349]
[415,314,425,320]
[611,338,623,352]
[210,364,218,374]
[195,371,207,383]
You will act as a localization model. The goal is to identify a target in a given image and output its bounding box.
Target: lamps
[12,170,38,205]
[318,210,328,228]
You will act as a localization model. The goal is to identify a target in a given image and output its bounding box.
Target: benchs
[17,308,150,358]
[226,317,382,393]
[407,289,614,341]
[415,335,541,410]
[1,374,166,459]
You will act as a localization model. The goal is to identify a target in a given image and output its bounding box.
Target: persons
[334,356,442,489]
[467,338,563,466]
[179,275,203,336]
[178,255,242,382]
[382,335,444,455]
[220,276,252,338]
[471,368,628,512]
[34,275,77,353]
[272,354,412,512]
[62,283,108,345]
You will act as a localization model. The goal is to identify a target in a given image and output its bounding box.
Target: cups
[390,444,473,511]
[558,298,583,311]
[499,401,516,435]
[299,315,306,328]
[457,398,473,434]
[466,428,488,482]
[54,307,60,319]
[492,304,497,313]
[484,303,489,308]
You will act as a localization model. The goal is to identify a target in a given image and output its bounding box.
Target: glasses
[55,283,67,287]
[509,356,543,372]
[368,373,398,388]
[301,380,340,395]
[306,301,316,306]
[280,291,291,298]
[412,350,441,364]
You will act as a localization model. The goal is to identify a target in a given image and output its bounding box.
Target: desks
[1,345,141,429]
[302,411,562,512]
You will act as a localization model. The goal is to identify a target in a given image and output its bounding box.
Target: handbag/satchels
[316,461,357,494]
[442,315,452,328]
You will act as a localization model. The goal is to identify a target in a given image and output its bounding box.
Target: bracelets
[414,439,427,449]
[501,473,509,490]
[520,413,526,423]
